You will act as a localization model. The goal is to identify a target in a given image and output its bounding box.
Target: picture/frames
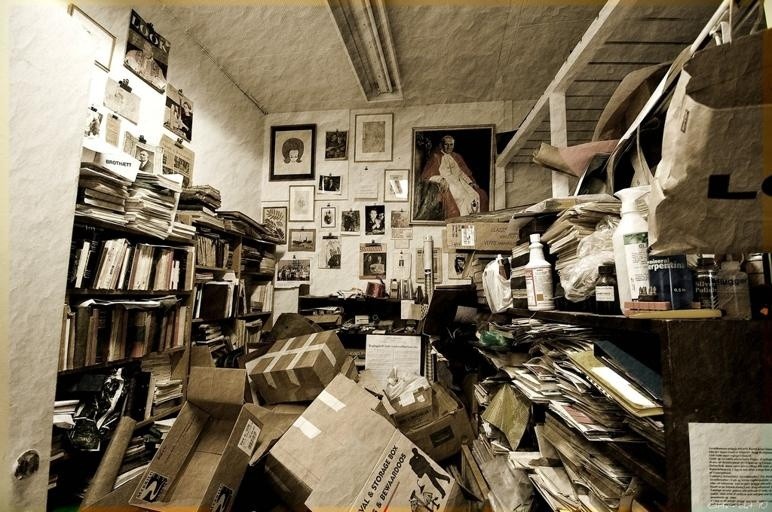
[321,207,336,228]
[288,185,315,222]
[68,3,117,73]
[409,123,496,226]
[287,228,316,252]
[262,206,287,244]
[353,112,393,162]
[384,169,409,203]
[269,123,317,180]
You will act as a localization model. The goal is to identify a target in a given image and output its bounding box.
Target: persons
[278,262,310,281]
[97,113,103,127]
[281,137,305,174]
[410,447,450,499]
[137,150,154,173]
[317,131,385,276]
[420,134,489,220]
[455,256,466,272]
[125,40,166,90]
[177,101,193,141]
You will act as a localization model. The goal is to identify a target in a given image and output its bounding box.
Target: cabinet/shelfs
[424,288,772,512]
[46,216,277,511]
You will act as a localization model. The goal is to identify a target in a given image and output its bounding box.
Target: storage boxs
[76,306,477,512]
[445,222,518,251]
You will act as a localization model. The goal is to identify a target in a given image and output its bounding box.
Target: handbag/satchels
[647,0,771,257]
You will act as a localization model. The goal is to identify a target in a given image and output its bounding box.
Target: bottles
[693,252,762,321]
[593,266,619,314]
[525,233,554,311]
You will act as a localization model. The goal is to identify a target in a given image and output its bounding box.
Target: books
[47,160,286,502]
[469,188,668,511]
